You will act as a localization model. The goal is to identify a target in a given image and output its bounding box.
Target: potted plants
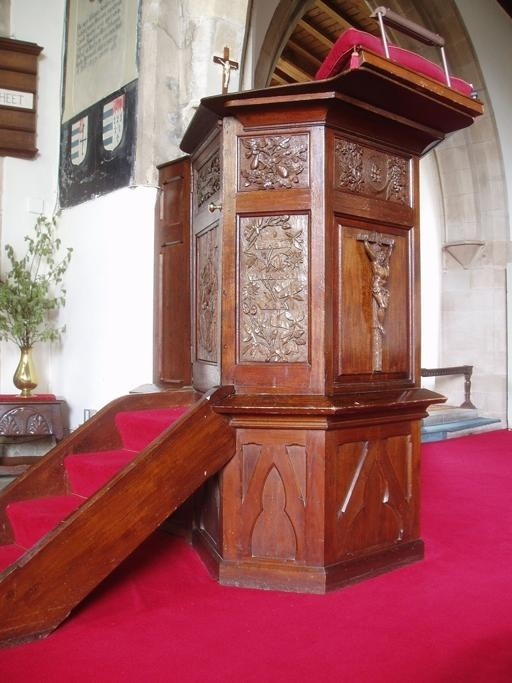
[0,212,76,395]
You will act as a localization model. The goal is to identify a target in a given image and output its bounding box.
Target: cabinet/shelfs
[0,395,66,477]
